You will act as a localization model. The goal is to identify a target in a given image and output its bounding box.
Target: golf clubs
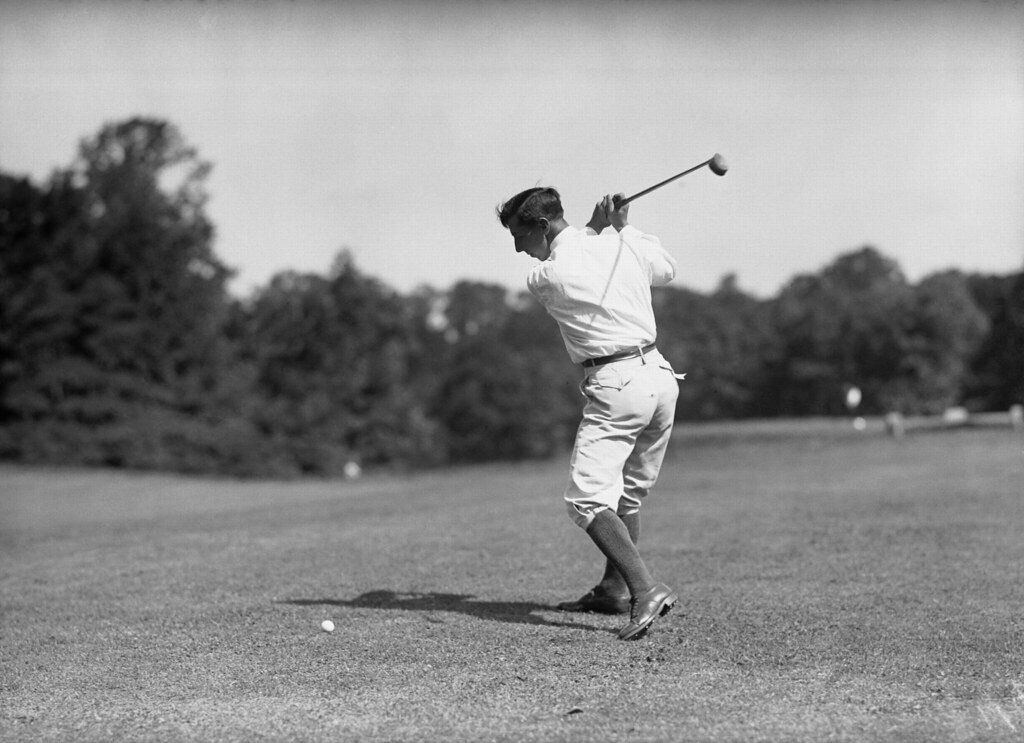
[615,153,728,208]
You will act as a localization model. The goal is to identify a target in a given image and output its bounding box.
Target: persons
[500,187,680,638]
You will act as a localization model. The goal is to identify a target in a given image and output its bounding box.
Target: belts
[585,341,658,365]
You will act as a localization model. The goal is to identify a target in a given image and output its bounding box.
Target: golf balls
[321,620,334,633]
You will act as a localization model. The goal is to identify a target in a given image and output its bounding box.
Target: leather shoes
[554,587,632,614]
[617,583,678,641]
